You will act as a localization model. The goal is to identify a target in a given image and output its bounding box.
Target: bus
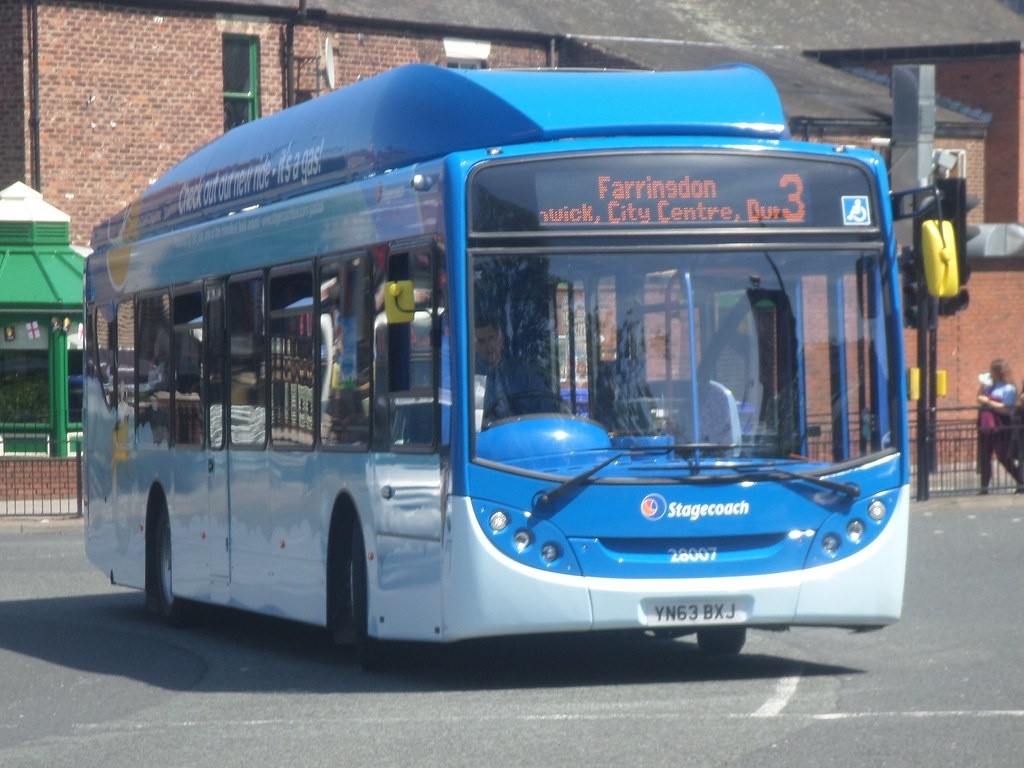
[82,60,962,670]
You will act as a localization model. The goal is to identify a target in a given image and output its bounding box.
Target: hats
[979,372,993,386]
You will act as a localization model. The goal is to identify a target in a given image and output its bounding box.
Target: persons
[975,357,1023,496]
[474,317,572,422]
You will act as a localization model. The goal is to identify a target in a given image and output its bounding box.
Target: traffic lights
[897,250,922,329]
[936,177,980,315]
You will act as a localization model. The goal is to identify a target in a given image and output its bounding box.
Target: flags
[4,324,16,342]
[26,319,41,340]
